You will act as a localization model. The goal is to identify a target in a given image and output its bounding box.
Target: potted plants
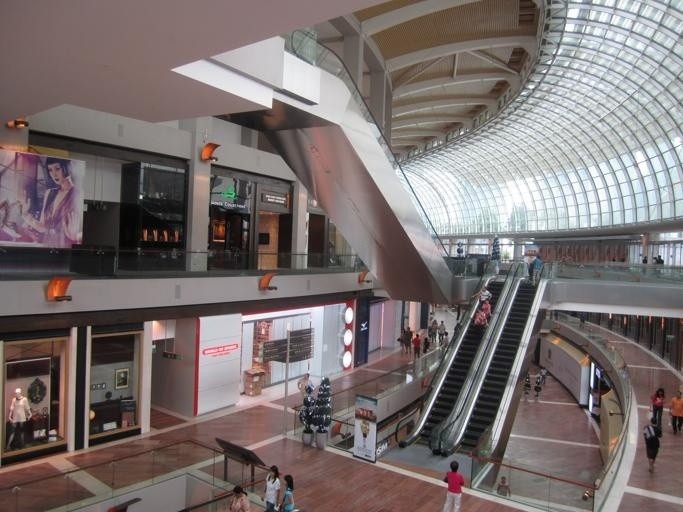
[298,384,315,446]
[491,237,501,274]
[312,376,333,449]
[523,372,531,404]
[533,375,542,404]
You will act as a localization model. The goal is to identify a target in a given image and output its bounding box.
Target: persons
[496,476,511,496]
[6,388,32,451]
[643,417,663,472]
[649,388,665,427]
[613,255,664,275]
[472,287,493,337]
[531,255,543,286]
[15,157,83,243]
[297,373,312,402]
[399,313,448,359]
[540,367,547,384]
[669,391,683,434]
[261,465,280,512]
[228,486,250,512]
[442,461,464,512]
[279,475,294,512]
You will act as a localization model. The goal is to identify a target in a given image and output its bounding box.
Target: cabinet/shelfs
[244,368,265,396]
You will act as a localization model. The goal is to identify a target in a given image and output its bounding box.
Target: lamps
[609,408,624,415]
[259,273,277,290]
[46,274,76,302]
[201,143,220,162]
[358,271,372,283]
[7,114,29,128]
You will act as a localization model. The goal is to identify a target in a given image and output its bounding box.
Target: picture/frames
[212,220,225,243]
[115,367,129,389]
